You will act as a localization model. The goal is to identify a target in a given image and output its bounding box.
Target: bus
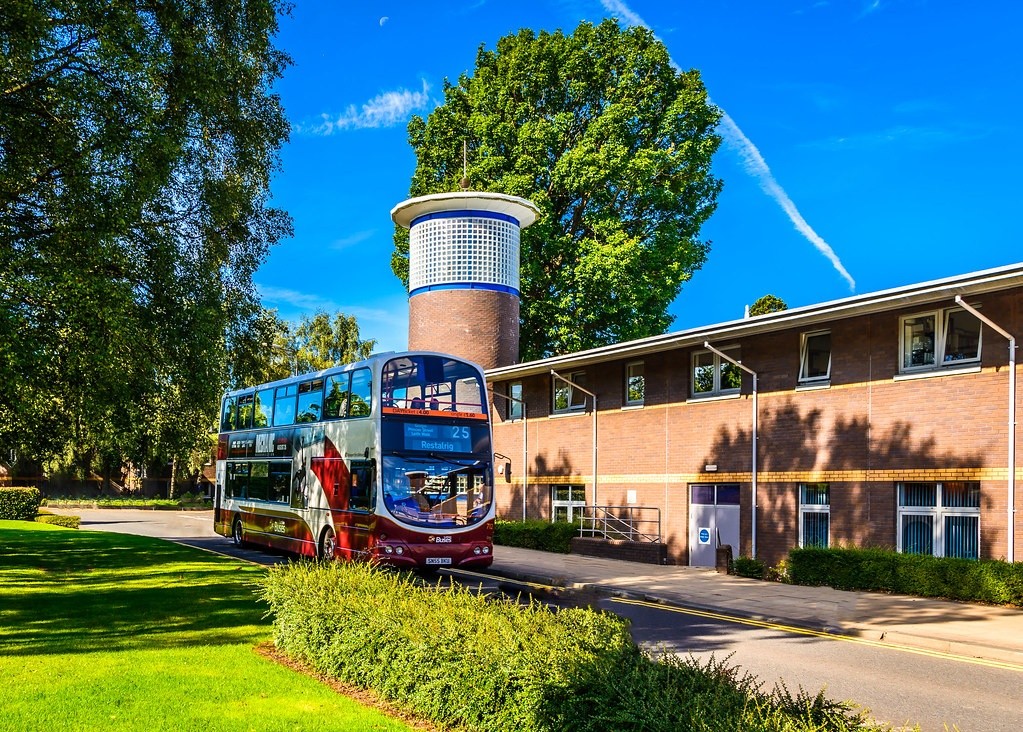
[212,349,494,573]
